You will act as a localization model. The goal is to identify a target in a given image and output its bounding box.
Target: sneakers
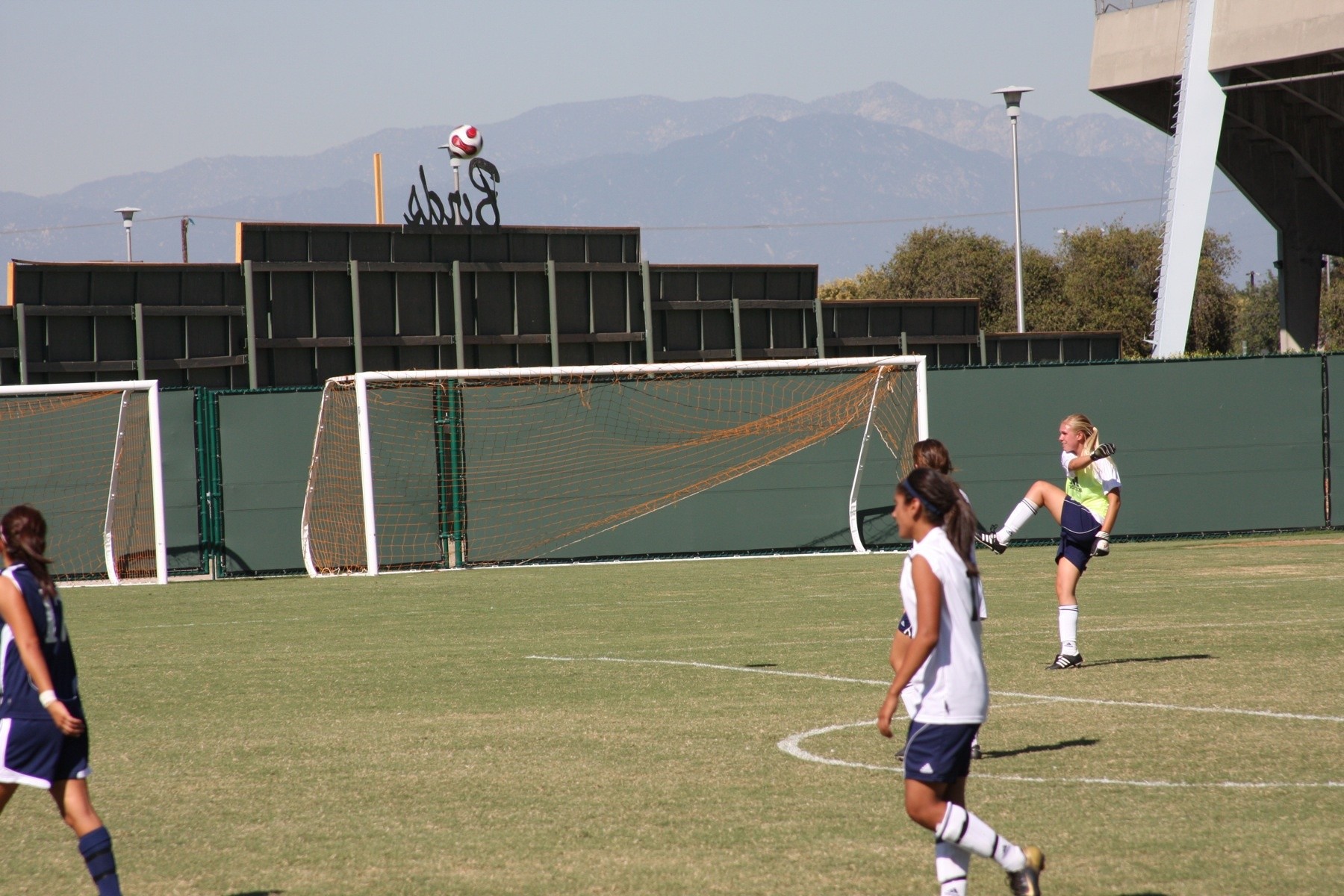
[1047,651,1084,671]
[1007,845,1045,895]
[972,524,1009,556]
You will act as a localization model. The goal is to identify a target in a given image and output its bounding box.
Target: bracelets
[39,689,56,709]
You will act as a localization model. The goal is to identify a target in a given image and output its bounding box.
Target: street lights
[436,144,464,225]
[991,85,1033,333]
[114,205,142,262]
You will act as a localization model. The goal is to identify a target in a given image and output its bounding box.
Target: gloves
[1090,443,1117,461]
[1090,531,1111,557]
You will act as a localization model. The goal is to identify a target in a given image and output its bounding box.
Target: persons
[876,439,1046,896]
[0,504,122,896]
[976,414,1122,672]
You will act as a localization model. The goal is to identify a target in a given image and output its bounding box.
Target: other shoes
[894,745,905,760]
[970,744,982,759]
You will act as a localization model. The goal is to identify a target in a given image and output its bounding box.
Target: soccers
[448,124,483,160]
